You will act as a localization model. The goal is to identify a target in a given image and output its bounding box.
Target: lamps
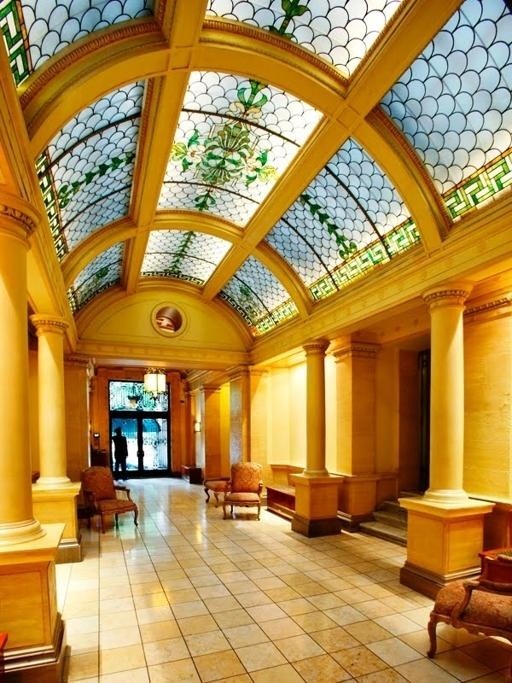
[144,368,165,395]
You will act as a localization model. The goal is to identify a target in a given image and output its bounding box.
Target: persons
[111,427,128,480]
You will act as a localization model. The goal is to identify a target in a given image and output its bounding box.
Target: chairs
[80,465,138,533]
[204,477,230,507]
[223,461,264,520]
[427,553,511,657]
